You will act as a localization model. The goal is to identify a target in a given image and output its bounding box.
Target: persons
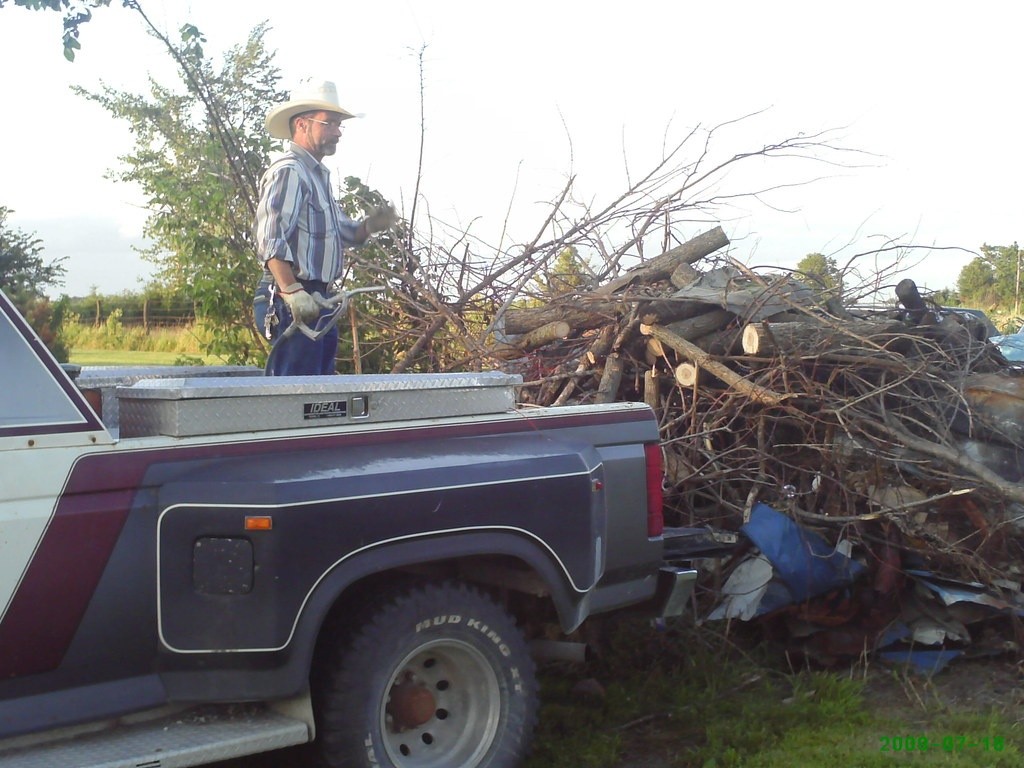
[253,80,399,376]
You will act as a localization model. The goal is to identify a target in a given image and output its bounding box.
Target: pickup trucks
[0,290,736,768]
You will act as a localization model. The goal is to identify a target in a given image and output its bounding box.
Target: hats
[265,82,365,138]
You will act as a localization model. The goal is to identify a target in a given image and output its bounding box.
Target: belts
[261,272,334,295]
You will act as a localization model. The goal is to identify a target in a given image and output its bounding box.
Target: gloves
[365,205,398,233]
[279,282,319,325]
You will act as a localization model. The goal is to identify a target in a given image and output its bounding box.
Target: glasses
[302,116,346,134]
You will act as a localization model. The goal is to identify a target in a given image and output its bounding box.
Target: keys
[264,307,279,340]
